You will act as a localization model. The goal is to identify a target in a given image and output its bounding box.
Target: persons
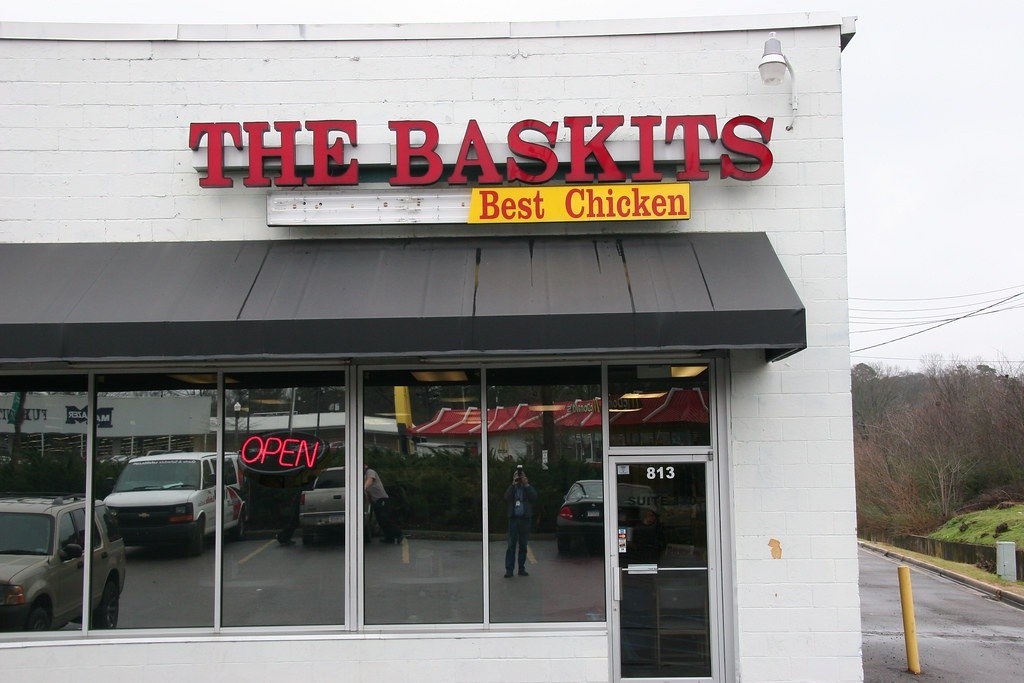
[463,442,478,456]
[504,471,538,577]
[364,464,404,544]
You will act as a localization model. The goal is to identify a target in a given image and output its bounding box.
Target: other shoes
[518,570,528,576]
[504,570,514,578]
[397,535,405,544]
[381,538,395,544]
[277,534,296,546]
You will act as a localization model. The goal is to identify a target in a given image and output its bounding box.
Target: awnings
[0,243,807,364]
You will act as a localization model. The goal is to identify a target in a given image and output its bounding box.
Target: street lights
[234,401,242,452]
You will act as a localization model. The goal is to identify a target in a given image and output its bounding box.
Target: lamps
[758,31,797,130]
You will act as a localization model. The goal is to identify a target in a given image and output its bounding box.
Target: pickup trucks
[300,466,382,543]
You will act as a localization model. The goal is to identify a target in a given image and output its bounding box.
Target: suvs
[0,491,125,632]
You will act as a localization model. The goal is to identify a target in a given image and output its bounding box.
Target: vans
[103,452,246,558]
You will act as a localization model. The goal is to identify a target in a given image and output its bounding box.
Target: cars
[554,480,665,559]
